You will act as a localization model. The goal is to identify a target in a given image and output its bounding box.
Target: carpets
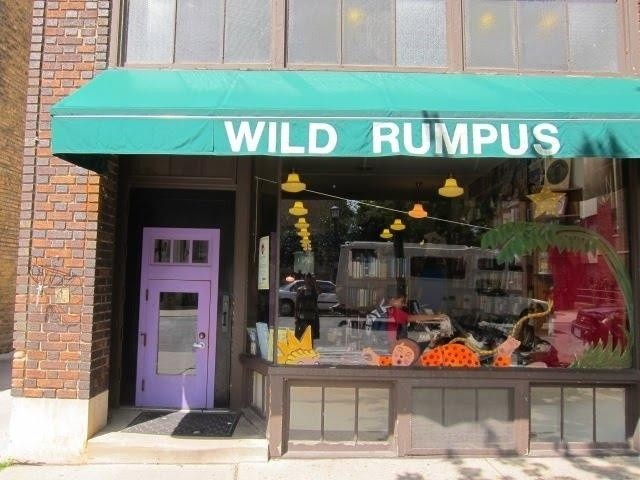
[121,411,241,437]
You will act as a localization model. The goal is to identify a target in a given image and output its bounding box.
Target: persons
[290,271,324,346]
[512,296,557,361]
[363,285,449,350]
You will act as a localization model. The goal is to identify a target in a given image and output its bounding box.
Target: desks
[338,306,450,351]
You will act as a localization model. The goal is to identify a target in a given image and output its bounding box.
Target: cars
[571,305,624,350]
[278,280,338,315]
[439,287,549,331]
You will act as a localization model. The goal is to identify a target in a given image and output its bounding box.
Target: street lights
[331,204,342,263]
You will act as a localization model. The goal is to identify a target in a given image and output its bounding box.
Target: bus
[334,241,524,326]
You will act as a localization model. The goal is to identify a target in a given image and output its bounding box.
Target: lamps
[438,171,465,199]
[408,181,429,218]
[280,169,313,252]
[380,228,392,240]
[389,218,407,231]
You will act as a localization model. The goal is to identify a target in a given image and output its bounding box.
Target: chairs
[448,296,554,366]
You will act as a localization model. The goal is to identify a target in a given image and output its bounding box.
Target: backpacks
[368,312,389,349]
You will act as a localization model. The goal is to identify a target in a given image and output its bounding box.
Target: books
[342,249,411,345]
[418,157,529,342]
